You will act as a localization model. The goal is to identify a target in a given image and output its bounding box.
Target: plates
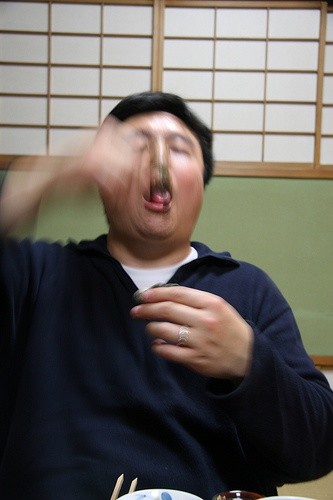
[112,489,202,499]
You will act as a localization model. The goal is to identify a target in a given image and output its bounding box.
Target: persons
[0,92,333,500]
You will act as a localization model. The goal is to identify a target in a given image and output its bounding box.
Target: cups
[213,490,261,500]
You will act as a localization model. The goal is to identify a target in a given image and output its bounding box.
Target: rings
[176,326,190,346]
